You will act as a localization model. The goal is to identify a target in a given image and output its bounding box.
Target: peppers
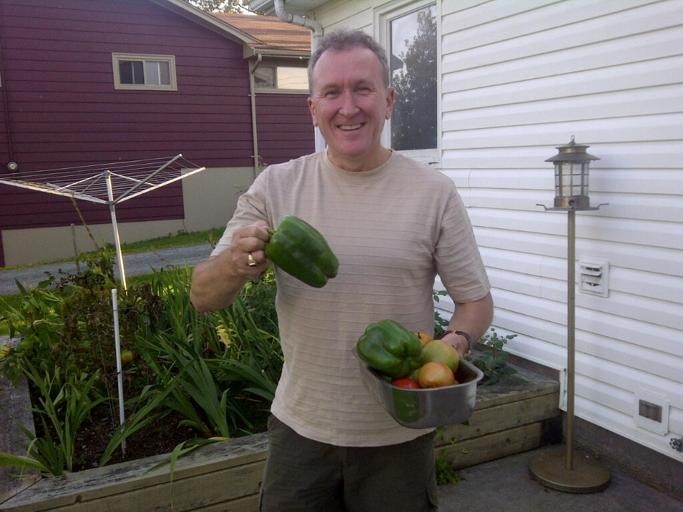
[263,216,340,290]
[356,318,423,379]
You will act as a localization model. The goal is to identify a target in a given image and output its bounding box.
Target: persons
[189,29,494,512]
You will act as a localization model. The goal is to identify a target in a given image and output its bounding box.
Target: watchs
[438,329,473,358]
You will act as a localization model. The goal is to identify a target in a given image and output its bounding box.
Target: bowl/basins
[352,346,484,430]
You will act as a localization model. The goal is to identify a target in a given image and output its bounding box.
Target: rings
[247,251,258,268]
